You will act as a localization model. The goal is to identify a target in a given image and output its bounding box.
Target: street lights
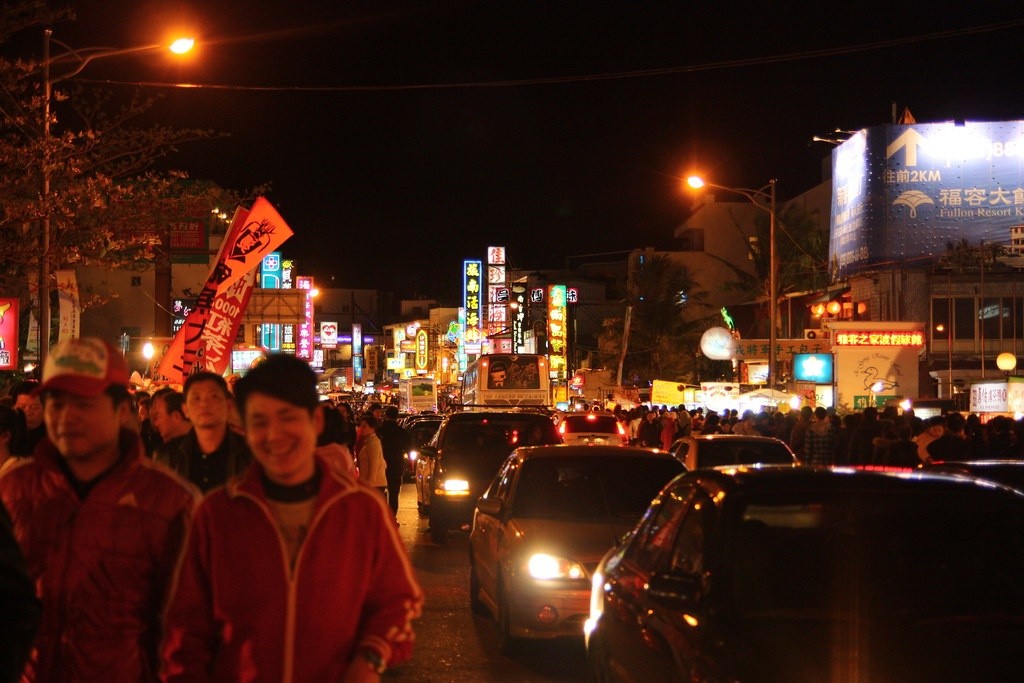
[39,38,199,373]
[688,178,776,386]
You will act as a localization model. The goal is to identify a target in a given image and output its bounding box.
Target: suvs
[553,410,629,449]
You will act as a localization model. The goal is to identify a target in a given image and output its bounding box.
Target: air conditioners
[804,329,824,339]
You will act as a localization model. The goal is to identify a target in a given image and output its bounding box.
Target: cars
[352,399,397,412]
[667,434,820,532]
[579,463,1024,683]
[468,445,689,652]
[414,411,560,545]
[394,411,445,480]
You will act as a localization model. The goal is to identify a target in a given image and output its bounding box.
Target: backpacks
[673,423,688,441]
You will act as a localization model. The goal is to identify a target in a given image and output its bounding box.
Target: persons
[0,331,423,683]
[584,394,1024,468]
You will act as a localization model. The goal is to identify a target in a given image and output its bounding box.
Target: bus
[328,392,352,404]
[399,377,437,413]
[462,353,551,412]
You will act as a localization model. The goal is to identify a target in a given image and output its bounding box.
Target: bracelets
[358,646,387,676]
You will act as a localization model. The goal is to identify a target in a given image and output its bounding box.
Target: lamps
[946,119,965,127]
[813,128,857,144]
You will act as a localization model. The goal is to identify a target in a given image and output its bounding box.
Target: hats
[35,337,129,398]
